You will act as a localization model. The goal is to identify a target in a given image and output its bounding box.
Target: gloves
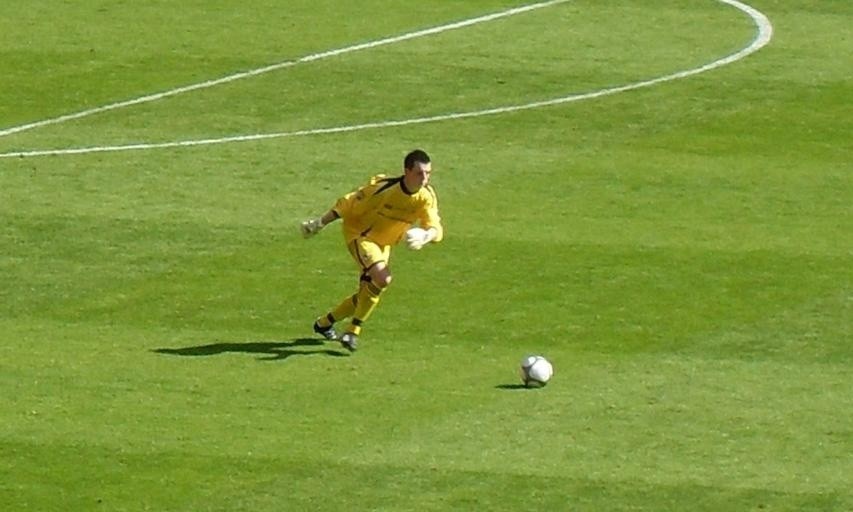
[300,217,324,239]
[404,227,438,252]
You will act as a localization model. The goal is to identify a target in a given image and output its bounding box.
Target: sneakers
[313,319,338,341]
[338,332,360,352]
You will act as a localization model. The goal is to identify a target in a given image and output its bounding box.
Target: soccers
[520,355,555,387]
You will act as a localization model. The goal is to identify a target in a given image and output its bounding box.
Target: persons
[299,150,443,355]
